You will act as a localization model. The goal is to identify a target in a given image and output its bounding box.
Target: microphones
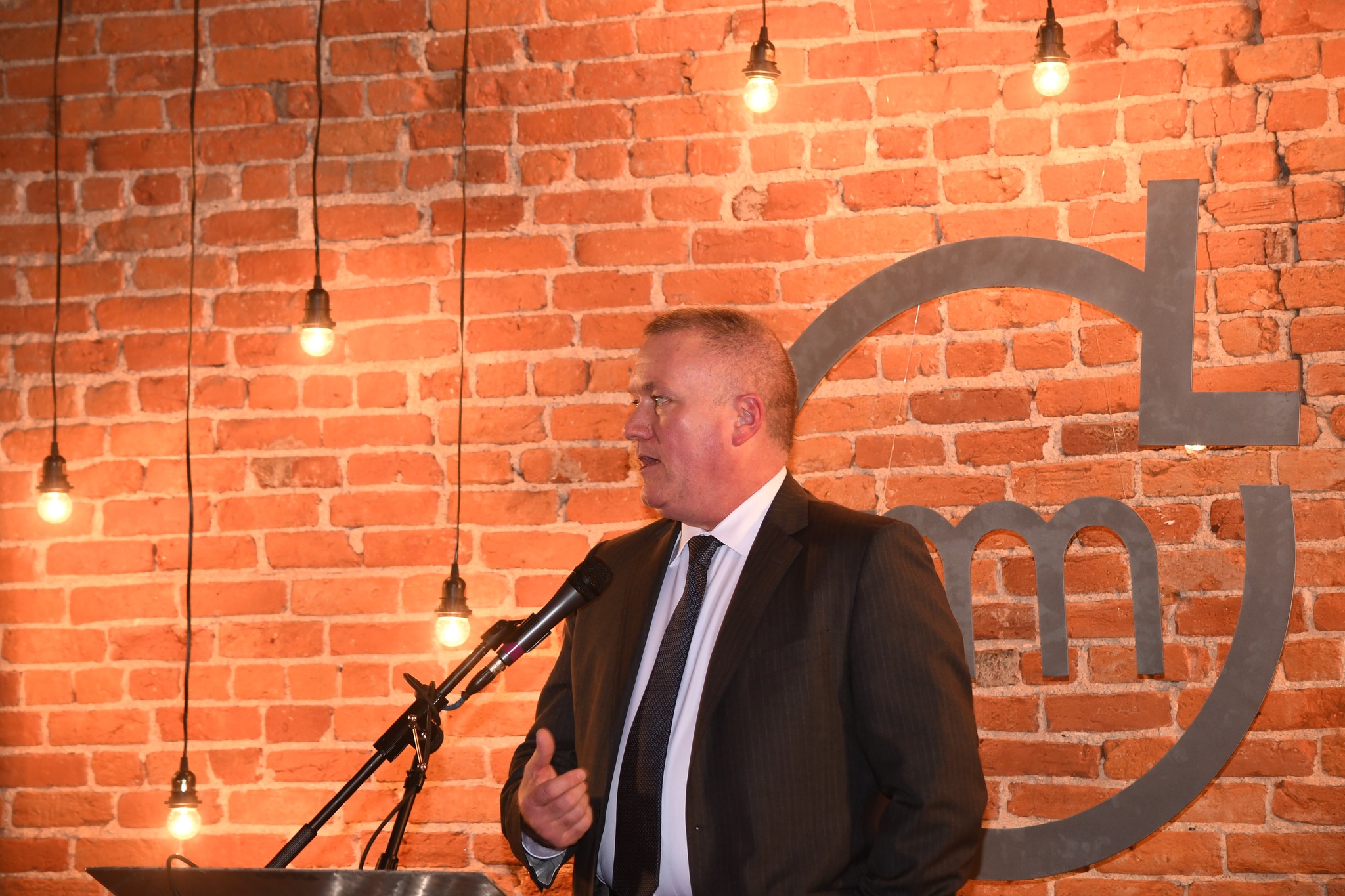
[466,557,614,700]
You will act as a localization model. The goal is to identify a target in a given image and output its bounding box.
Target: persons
[495,305,991,896]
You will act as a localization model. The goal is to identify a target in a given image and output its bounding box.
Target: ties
[610,534,724,896]
